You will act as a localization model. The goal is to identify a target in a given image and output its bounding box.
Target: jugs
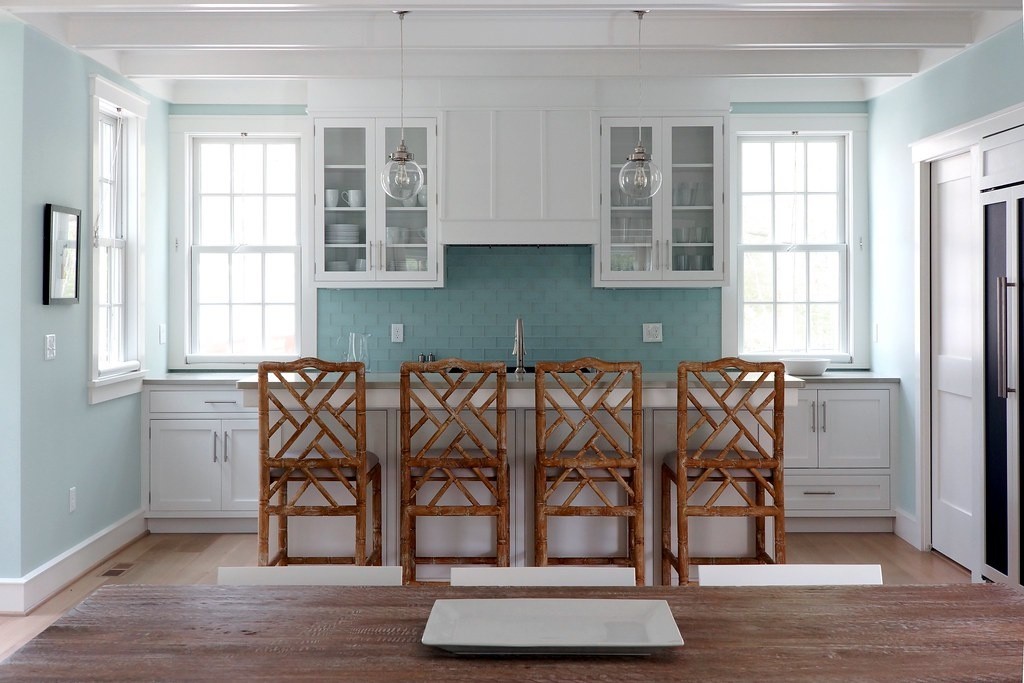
[335,332,373,373]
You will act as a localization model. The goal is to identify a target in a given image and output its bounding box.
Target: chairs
[661,357,786,587]
[399,358,510,587]
[258,356,381,566]
[535,355,646,586]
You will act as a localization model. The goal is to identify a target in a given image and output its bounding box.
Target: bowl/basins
[778,357,831,376]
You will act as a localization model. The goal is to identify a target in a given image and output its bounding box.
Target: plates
[389,256,429,271]
[324,224,367,271]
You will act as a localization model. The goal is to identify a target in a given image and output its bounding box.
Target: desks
[0,580,1023,683]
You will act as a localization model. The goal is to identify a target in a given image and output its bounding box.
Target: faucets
[511,317,527,375]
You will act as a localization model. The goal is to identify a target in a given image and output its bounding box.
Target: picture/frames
[42,203,84,306]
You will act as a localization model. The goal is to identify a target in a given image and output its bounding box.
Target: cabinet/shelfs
[313,112,444,288]
[140,388,282,519]
[591,112,732,288]
[756,384,897,517]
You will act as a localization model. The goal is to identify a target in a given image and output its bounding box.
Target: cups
[341,190,365,207]
[609,181,715,272]
[402,190,418,206]
[399,227,410,244]
[417,185,427,207]
[325,189,339,207]
[418,226,427,242]
[386,226,401,244]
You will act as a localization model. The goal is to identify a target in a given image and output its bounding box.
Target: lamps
[618,11,660,198]
[381,7,423,202]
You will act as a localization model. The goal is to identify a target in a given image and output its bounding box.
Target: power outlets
[68,485,78,513]
[391,323,403,342]
[45,334,58,360]
[643,321,663,342]
